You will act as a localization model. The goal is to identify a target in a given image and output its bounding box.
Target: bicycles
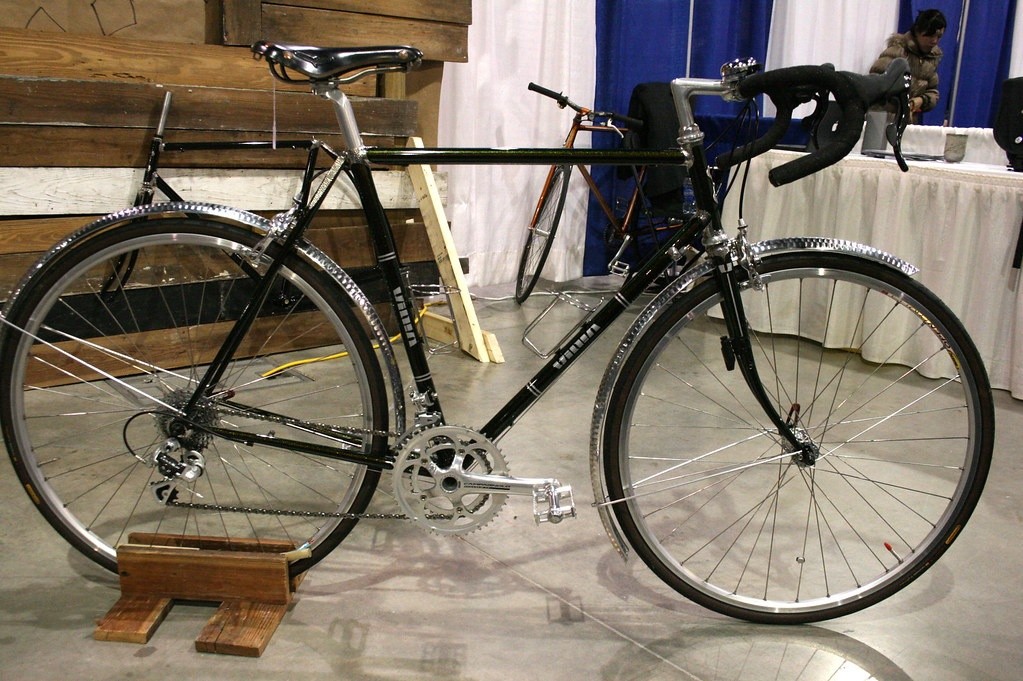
[3,42,997,624]
[513,80,726,306]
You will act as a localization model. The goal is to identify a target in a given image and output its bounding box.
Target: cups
[944,133,969,164]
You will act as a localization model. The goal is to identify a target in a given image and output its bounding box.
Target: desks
[706,144,1023,402]
[862,120,1010,165]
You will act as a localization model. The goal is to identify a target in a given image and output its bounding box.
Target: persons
[867,9,947,125]
